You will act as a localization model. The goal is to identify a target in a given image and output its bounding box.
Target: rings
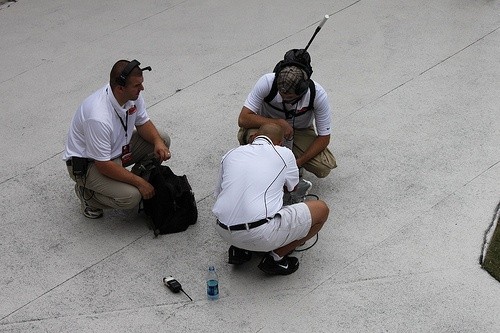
[167,152,170,155]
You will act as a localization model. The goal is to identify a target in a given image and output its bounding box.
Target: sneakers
[227,245,253,265]
[74,183,103,219]
[258,252,299,277]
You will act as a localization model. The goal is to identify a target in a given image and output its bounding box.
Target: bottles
[206,265,219,301]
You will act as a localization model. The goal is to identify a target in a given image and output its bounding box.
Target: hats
[277,65,307,97]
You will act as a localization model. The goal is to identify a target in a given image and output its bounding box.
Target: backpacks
[138,163,198,238]
[264,60,316,117]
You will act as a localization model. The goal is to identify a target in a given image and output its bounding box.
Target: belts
[216,217,272,232]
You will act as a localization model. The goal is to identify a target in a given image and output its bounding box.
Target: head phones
[115,60,141,87]
[294,77,310,96]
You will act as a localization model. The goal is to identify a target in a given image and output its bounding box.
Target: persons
[237,48,338,180]
[211,122,329,276]
[63,59,171,219]
